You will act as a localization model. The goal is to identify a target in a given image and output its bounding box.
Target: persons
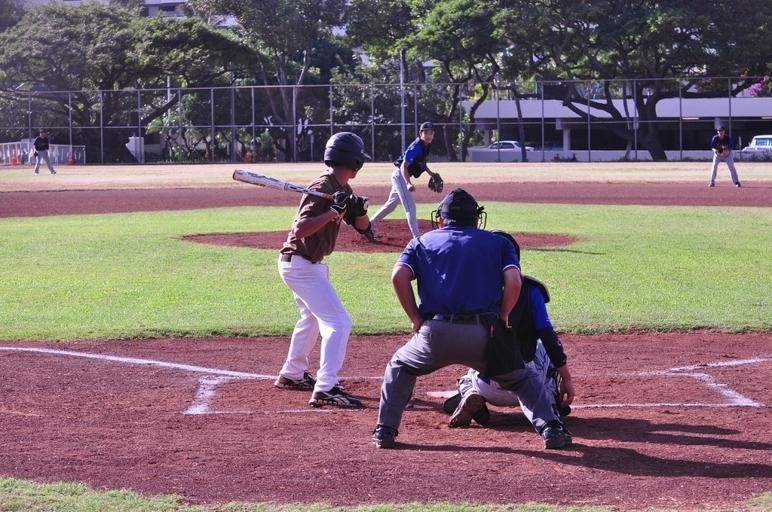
[706,125,741,189]
[272,127,373,410]
[31,128,57,176]
[371,187,574,450]
[442,228,576,432]
[366,120,442,243]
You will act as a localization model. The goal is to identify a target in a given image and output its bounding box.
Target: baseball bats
[232,170,368,210]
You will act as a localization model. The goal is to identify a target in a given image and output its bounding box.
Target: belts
[428,314,483,325]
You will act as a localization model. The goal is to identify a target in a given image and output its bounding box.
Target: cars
[487,141,536,151]
[743,134,772,151]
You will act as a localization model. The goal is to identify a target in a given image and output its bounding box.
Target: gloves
[330,191,350,216]
[349,194,368,217]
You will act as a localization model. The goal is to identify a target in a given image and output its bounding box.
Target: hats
[717,126,725,131]
[420,121,435,132]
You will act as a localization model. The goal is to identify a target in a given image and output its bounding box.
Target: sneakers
[736,182,741,187]
[309,386,365,409]
[274,372,315,390]
[448,392,484,427]
[709,183,715,187]
[542,424,572,448]
[373,427,395,448]
[366,231,378,242]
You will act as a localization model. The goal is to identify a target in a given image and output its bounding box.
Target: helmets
[431,188,488,230]
[323,131,372,172]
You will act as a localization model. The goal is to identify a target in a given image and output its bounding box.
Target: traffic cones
[66,151,74,165]
[10,150,18,164]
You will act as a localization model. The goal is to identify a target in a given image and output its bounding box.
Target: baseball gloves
[721,146,730,157]
[428,173,444,193]
